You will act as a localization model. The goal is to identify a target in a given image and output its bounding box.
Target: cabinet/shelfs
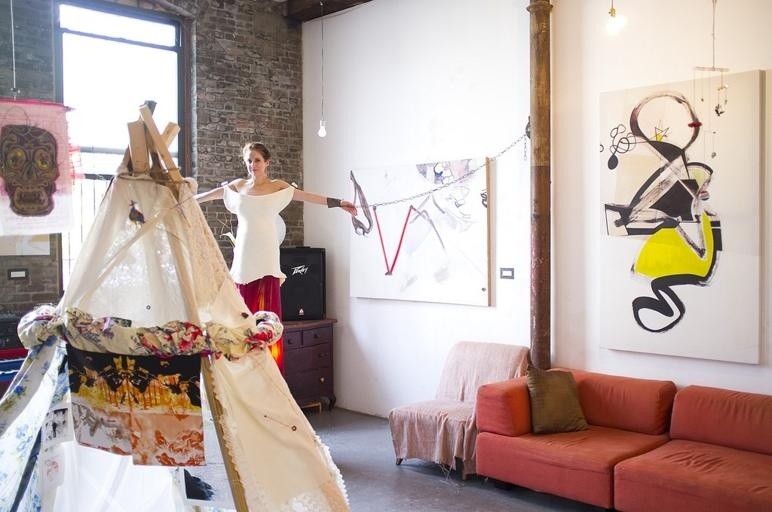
[280,317,338,414]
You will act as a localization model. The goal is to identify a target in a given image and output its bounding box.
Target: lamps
[316,0,328,137]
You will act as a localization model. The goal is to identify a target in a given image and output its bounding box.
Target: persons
[192,141,358,381]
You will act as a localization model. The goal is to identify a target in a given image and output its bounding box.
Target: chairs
[389,340,530,482]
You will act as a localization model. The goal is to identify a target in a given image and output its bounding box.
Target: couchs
[472,364,772,512]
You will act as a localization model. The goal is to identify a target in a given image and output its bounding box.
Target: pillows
[523,349,591,435]
